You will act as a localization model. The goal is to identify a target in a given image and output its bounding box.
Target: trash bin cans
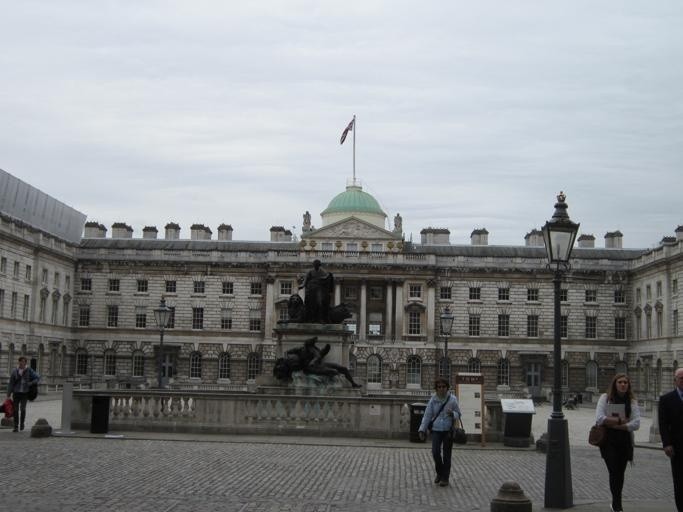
[91,396,109,433]
[410,403,426,443]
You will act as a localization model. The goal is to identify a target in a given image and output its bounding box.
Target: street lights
[154,294,171,386]
[438,304,455,377]
[540,191,582,509]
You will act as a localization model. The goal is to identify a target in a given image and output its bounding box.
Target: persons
[596,373,641,512]
[284,336,364,389]
[6,357,41,433]
[418,376,462,487]
[297,259,331,324]
[657,367,682,511]
[393,212,402,233]
[562,390,583,410]
[302,210,311,231]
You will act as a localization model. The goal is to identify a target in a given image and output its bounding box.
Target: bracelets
[618,416,621,426]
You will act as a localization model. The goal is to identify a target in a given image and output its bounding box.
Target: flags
[340,115,355,145]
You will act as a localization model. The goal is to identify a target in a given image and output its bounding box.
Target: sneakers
[434,475,449,486]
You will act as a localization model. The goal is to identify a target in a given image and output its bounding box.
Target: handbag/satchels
[427,420,434,432]
[0,398,15,418]
[586,424,609,447]
[27,377,37,402]
[449,424,467,445]
